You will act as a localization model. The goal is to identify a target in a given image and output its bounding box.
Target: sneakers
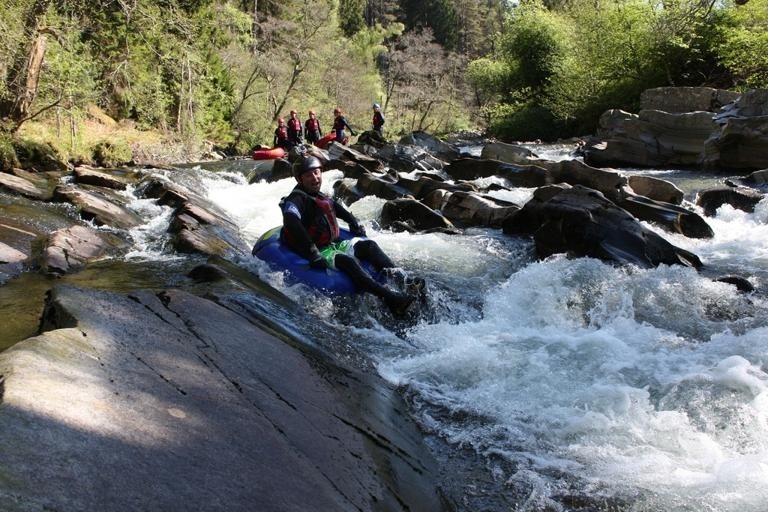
[407,277,425,293]
[392,294,417,320]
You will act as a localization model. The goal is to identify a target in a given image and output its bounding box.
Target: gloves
[347,221,365,237]
[307,254,331,270]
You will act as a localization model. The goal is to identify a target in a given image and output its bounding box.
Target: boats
[252,225,387,297]
[316,132,348,148]
[253,147,285,160]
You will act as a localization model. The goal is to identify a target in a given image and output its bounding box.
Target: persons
[372,103,386,133]
[332,108,356,143]
[273,117,289,147]
[288,111,303,147]
[303,109,322,146]
[278,154,426,321]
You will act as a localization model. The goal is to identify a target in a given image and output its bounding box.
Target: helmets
[277,117,285,123]
[309,110,315,115]
[292,154,323,176]
[290,110,297,115]
[333,108,342,115]
[373,104,380,109]
